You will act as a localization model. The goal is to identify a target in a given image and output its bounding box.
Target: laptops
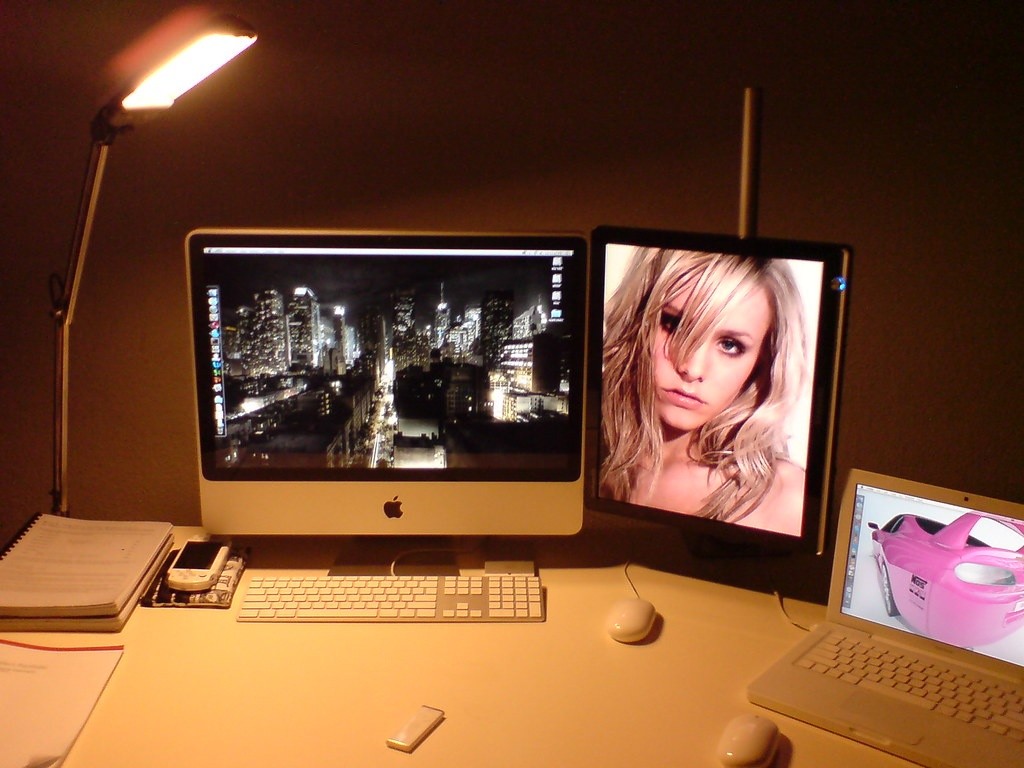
[746,466,1023,768]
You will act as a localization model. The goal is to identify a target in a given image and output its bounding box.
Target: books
[0,639,124,768]
[1,512,174,630]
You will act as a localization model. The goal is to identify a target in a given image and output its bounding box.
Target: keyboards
[237,577,546,624]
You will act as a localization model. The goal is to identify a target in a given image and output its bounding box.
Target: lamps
[44,3,256,513]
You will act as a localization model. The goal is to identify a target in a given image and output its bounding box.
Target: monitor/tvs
[584,229,854,566]
[183,227,591,583]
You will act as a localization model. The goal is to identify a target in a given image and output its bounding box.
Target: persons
[600,248,806,537]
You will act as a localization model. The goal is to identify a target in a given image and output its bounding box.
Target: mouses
[609,599,656,643]
[718,712,780,768]
